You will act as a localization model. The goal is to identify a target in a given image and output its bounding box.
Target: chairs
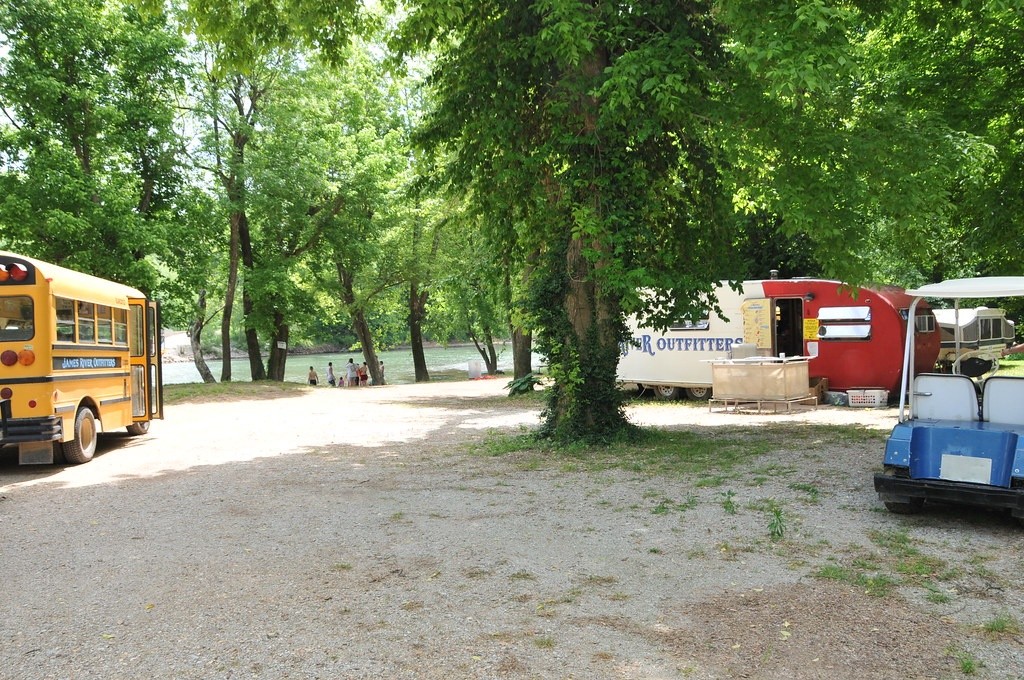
[730,343,757,358]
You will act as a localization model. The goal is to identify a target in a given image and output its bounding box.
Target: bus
[0,248,164,464]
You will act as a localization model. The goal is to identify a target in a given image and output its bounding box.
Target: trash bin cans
[467,359,482,379]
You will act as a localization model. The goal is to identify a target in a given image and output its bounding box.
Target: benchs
[536,365,548,374]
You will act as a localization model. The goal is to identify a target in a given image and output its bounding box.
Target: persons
[379,361,384,377]
[338,377,344,387]
[360,362,368,386]
[307,366,319,386]
[346,358,360,388]
[327,362,336,386]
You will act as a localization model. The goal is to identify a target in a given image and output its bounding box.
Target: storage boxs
[825,391,848,406]
[799,377,828,405]
[846,389,890,408]
